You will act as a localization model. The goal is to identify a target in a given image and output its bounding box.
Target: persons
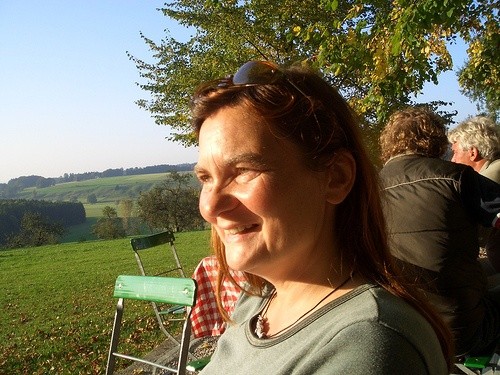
[189,60,449,375]
[370,106,500,359]
[445,114,499,184]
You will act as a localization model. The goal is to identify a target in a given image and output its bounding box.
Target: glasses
[218,59,314,112]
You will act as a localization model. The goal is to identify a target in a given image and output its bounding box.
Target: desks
[185,254,270,337]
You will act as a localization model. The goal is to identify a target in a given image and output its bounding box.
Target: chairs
[130,232,212,374]
[105,274,199,375]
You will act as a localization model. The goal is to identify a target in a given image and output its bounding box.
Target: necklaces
[253,261,359,339]
